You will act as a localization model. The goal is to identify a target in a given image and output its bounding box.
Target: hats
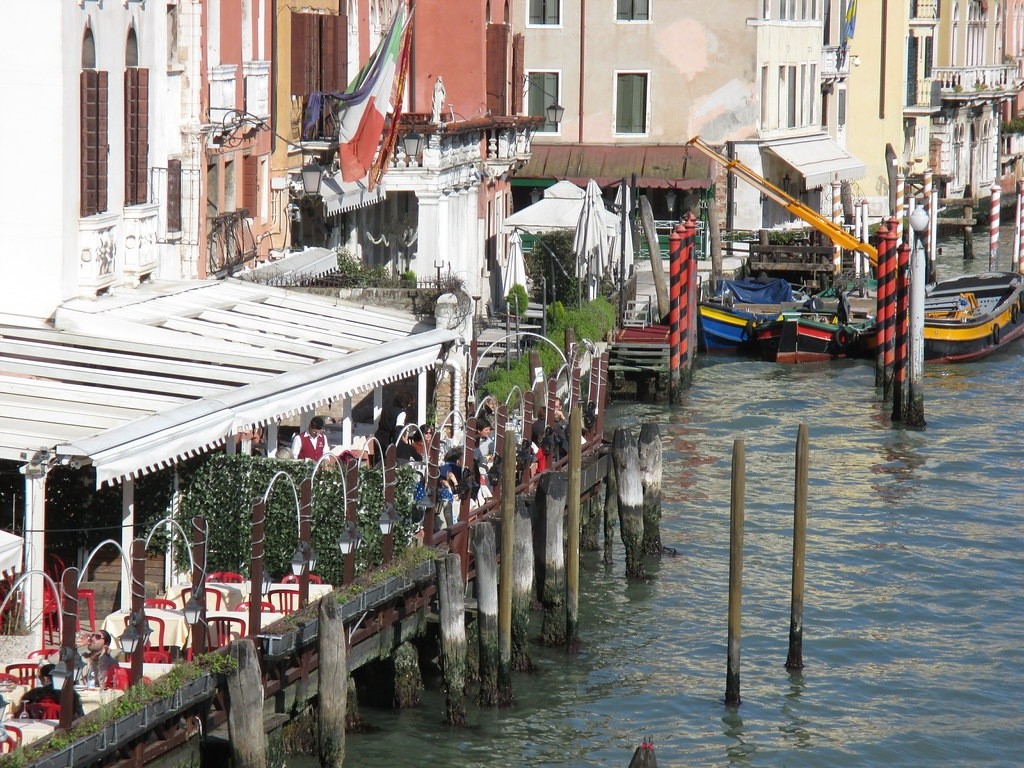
[99,629,111,646]
[39,664,56,677]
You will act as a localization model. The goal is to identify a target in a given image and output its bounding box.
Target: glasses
[424,432,432,435]
[92,634,104,640]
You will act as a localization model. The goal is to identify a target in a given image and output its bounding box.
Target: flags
[302,2,415,193]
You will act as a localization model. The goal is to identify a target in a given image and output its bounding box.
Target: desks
[2,581,333,750]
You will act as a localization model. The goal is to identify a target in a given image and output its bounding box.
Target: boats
[696,271,909,365]
[919,269,1024,365]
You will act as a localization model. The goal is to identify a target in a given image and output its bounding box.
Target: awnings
[762,136,866,191]
[510,143,718,192]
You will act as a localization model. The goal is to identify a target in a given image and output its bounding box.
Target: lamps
[300,150,322,209]
[402,120,421,156]
[522,73,564,124]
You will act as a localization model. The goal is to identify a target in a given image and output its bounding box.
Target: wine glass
[5,700,14,721]
[98,671,107,694]
[19,700,29,724]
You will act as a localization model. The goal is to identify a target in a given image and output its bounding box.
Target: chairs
[0,571,320,757]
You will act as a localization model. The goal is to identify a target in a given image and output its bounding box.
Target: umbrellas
[500,179,634,309]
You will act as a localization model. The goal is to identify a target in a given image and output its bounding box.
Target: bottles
[512,413,518,426]
[37,659,50,687]
[87,660,96,688]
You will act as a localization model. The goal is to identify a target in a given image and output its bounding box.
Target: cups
[515,434,522,444]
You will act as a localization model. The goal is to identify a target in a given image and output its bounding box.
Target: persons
[291,416,330,465]
[431,76,446,123]
[74,629,119,687]
[414,398,502,533]
[515,397,595,488]
[374,392,431,464]
[16,664,84,723]
